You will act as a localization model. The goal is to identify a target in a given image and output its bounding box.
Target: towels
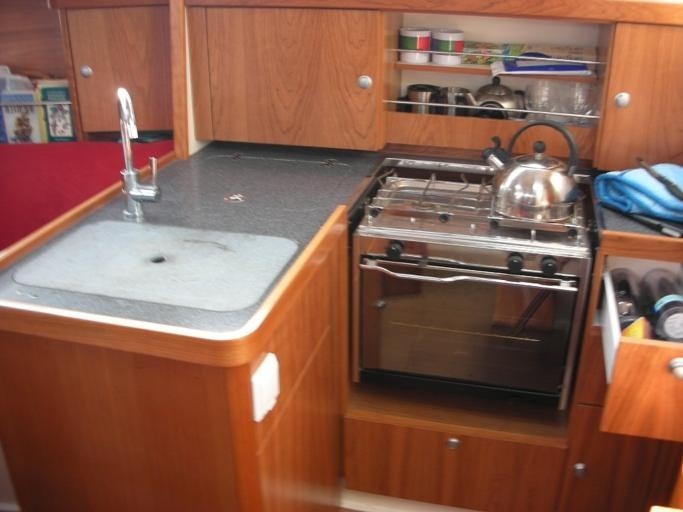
[593,161,683,222]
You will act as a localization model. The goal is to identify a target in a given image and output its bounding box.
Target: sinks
[10,221,298,312]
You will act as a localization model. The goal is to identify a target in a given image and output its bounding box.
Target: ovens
[350,229,592,410]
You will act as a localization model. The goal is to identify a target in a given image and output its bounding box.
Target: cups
[399,26,430,66]
[432,30,466,66]
[406,84,470,117]
[524,79,598,123]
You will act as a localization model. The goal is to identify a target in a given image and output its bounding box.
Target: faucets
[116,87,139,169]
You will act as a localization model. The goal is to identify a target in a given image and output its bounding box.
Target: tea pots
[464,76,525,118]
[481,120,580,222]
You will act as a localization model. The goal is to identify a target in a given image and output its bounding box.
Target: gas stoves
[354,168,594,249]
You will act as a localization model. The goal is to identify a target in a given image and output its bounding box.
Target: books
[0,63,75,145]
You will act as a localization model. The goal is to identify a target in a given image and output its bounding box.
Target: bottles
[610,266,683,341]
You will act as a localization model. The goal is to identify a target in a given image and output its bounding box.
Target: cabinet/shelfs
[338,406,571,512]
[0,219,352,511]
[556,230,682,512]
